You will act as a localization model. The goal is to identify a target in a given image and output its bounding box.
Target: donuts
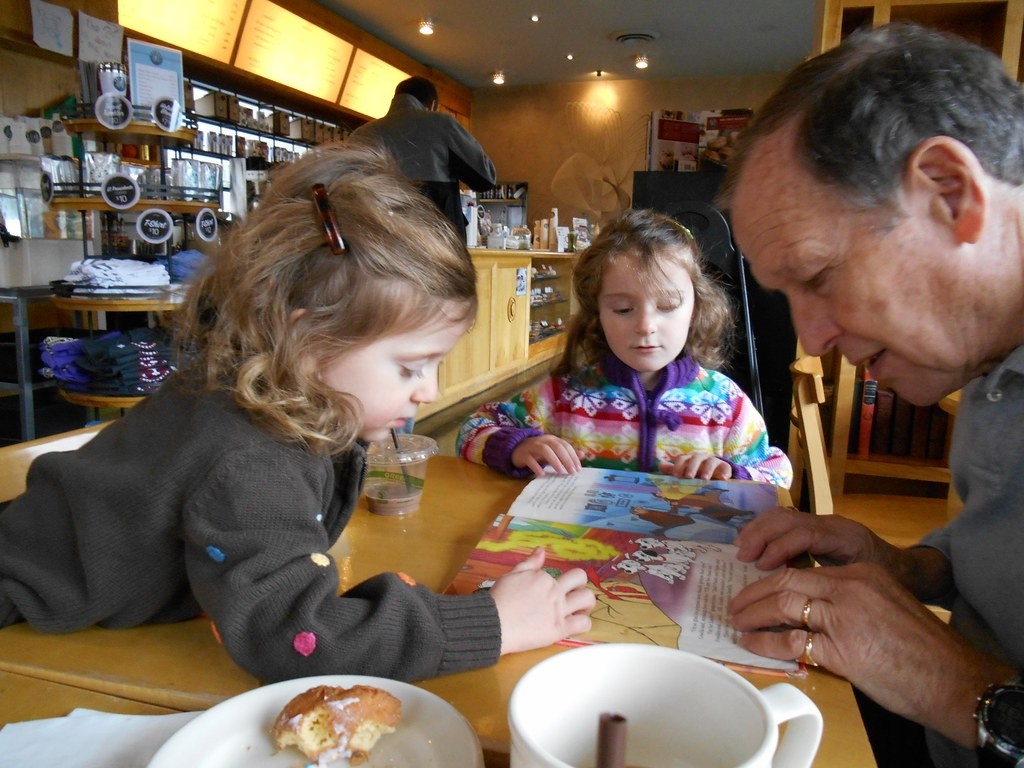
[658,149,674,169]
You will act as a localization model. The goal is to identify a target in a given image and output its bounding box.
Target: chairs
[791,355,834,515]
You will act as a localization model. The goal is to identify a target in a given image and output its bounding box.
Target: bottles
[119,218,128,250]
[482,184,512,199]
[110,220,118,252]
[101,214,109,254]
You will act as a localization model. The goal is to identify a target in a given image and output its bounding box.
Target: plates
[148,674,485,768]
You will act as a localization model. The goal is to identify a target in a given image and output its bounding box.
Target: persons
[0,143,598,681]
[347,76,496,245]
[454,210,792,490]
[714,21,1024,768]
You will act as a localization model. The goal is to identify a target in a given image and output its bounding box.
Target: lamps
[636,54,648,68]
[491,70,505,84]
[419,17,435,35]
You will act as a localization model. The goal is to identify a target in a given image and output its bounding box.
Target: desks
[0,452,872,768]
[0,668,192,768]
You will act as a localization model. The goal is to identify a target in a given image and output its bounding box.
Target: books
[858,367,946,460]
[447,464,806,679]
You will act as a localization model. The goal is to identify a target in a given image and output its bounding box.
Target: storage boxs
[183,80,352,167]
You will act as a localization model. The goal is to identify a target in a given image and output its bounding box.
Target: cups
[365,434,437,515]
[534,208,558,251]
[508,643,823,767]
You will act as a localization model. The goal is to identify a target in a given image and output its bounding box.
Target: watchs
[973,672,1024,768]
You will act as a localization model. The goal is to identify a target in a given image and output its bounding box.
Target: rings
[795,632,818,667]
[803,598,812,631]
[786,506,800,512]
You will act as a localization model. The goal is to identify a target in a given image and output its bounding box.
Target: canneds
[481,185,503,199]
[194,131,235,155]
[270,146,300,164]
[507,184,513,199]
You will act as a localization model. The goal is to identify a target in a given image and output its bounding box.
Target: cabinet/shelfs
[444,268,528,387]
[791,0,1024,509]
[47,119,224,408]
[530,273,571,345]
[162,83,352,169]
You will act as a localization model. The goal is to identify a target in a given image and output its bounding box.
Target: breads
[274,685,401,763]
[703,131,742,164]
